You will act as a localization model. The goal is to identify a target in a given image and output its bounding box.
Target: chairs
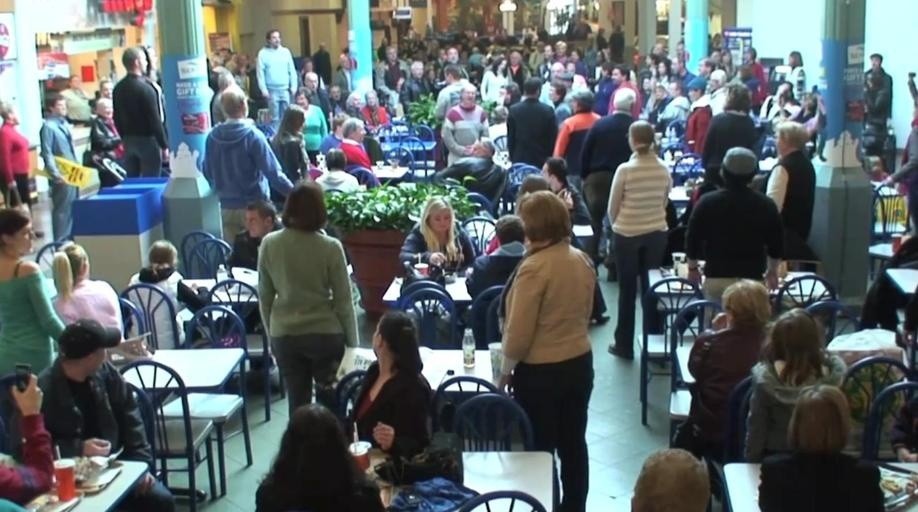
[0,96,917,512]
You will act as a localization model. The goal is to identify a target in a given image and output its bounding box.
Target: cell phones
[15,363,31,392]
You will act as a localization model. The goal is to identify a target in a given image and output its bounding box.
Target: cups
[488,342,502,371]
[415,262,429,276]
[671,252,706,281]
[890,233,902,254]
[52,459,77,501]
[376,161,384,171]
[347,440,372,471]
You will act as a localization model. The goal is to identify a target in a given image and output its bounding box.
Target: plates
[23,466,121,511]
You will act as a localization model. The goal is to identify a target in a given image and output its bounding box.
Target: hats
[722,147,758,178]
[58,318,121,360]
[687,77,707,91]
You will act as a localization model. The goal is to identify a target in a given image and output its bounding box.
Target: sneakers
[609,344,634,360]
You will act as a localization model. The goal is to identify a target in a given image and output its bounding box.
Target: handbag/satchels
[369,427,466,485]
[79,148,117,174]
[379,474,482,512]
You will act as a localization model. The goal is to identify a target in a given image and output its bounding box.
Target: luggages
[860,130,899,175]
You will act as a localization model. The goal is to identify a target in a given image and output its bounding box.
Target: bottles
[460,327,477,368]
[215,263,228,291]
[653,125,684,166]
